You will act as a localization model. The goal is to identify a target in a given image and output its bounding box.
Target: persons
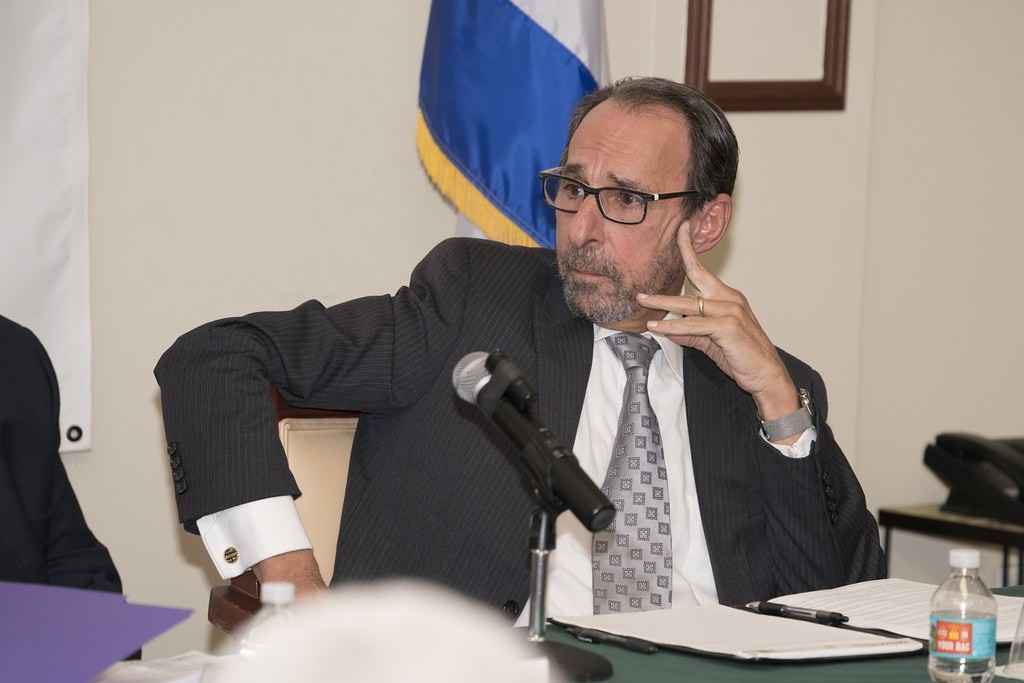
[151,72,894,617]
[2,311,145,663]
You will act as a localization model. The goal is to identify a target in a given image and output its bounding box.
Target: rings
[698,295,704,317]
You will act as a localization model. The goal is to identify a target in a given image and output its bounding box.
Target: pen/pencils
[745,601,850,626]
[566,628,660,656]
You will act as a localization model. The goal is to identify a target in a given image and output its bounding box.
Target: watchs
[755,387,815,441]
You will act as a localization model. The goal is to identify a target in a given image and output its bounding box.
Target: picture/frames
[682,0,852,112]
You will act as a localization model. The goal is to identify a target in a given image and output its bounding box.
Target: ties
[593,331,672,619]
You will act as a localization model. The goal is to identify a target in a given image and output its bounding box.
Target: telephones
[923,432,1024,527]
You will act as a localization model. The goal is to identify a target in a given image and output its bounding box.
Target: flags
[414,0,615,253]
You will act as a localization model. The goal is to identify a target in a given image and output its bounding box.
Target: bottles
[923,547,999,682]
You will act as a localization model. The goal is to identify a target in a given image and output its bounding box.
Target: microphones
[452,352,618,534]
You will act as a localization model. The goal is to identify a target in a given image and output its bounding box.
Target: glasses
[538,165,712,225]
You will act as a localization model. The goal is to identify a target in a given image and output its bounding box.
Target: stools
[878,500,1024,588]
